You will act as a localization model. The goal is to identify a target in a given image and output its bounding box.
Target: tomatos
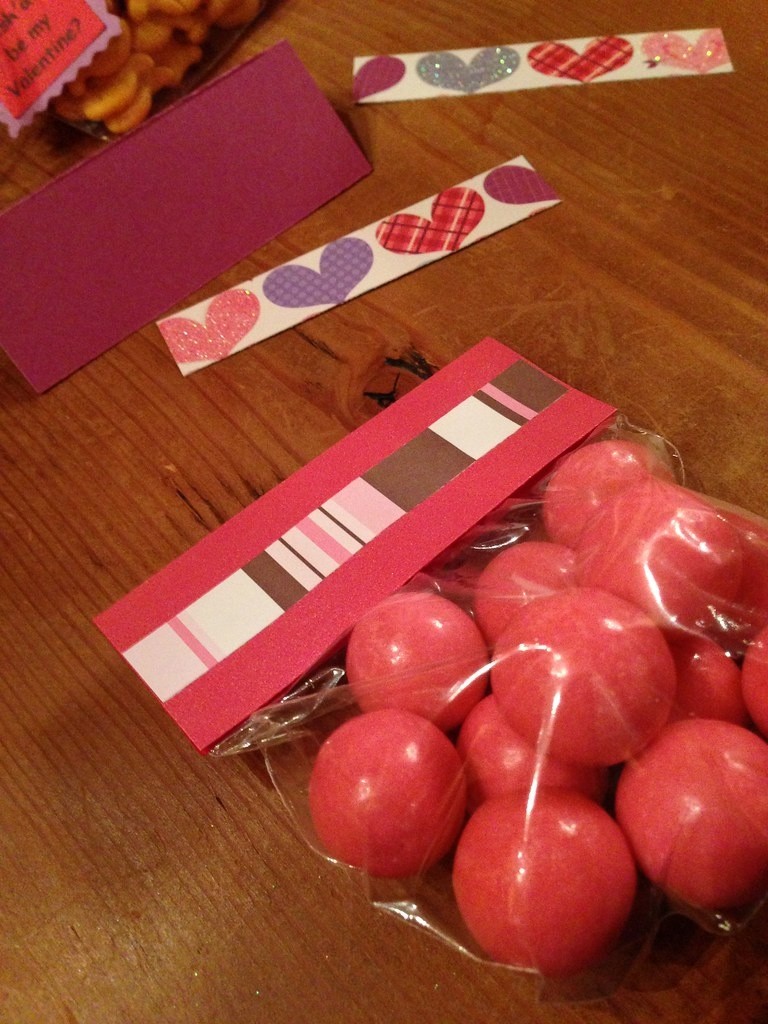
[307,441,768,971]
